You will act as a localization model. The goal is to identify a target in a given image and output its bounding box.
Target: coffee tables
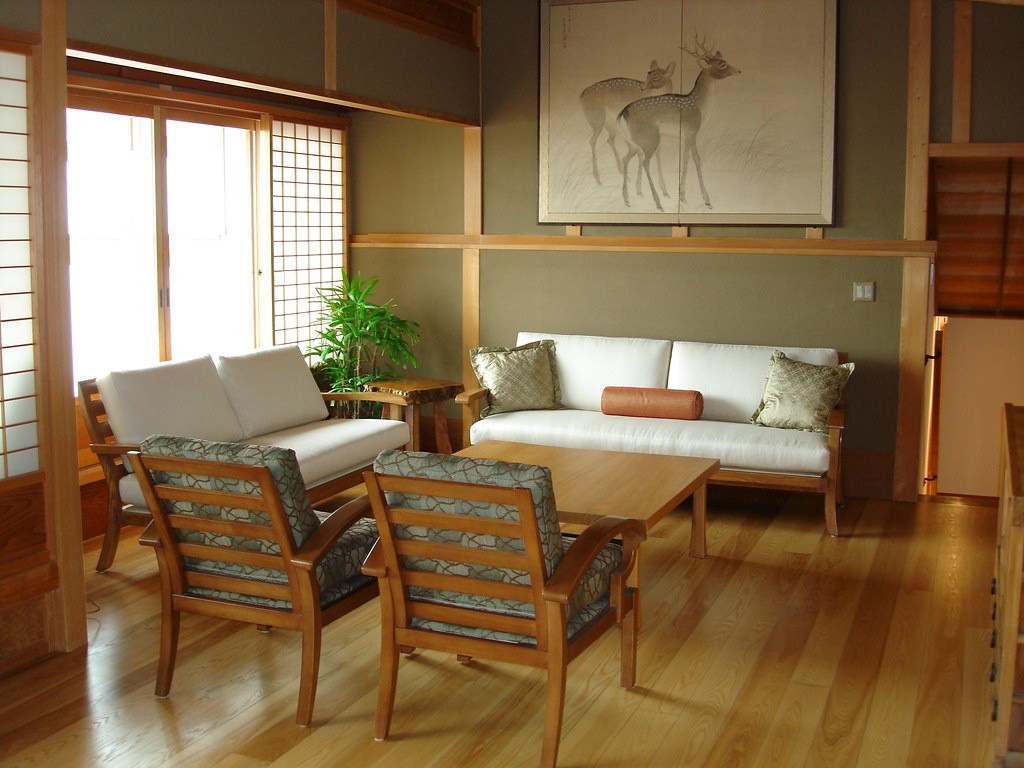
[450,439,721,629]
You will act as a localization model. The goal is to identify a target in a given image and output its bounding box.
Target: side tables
[364,378,465,456]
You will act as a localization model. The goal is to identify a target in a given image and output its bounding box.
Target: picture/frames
[538,1,836,226]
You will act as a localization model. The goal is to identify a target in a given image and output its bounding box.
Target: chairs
[361,447,649,767]
[126,434,417,727]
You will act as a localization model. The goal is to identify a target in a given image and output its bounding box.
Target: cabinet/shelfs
[988,404,1024,767]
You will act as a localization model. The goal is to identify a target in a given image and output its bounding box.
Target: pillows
[469,339,566,419]
[748,347,855,433]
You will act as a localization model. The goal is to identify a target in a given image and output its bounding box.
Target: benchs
[77,343,411,573]
[455,331,856,536]
